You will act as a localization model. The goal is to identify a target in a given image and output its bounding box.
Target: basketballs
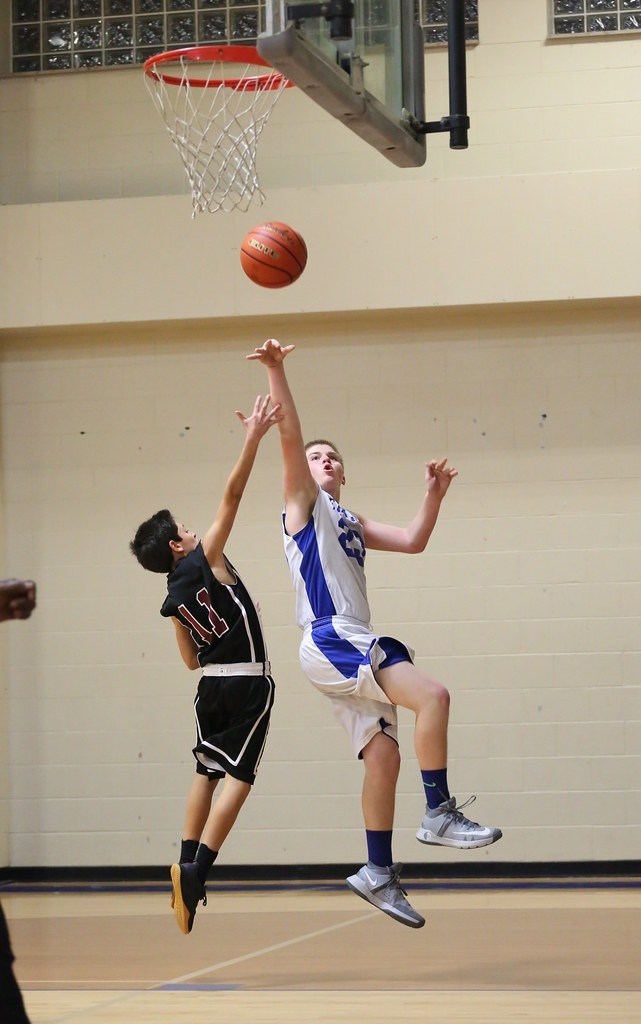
[240,221,309,289]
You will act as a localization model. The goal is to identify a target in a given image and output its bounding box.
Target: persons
[131,392,285,936]
[244,338,505,928]
[0,577,36,1024]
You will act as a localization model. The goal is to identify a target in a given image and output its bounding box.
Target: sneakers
[416,795,502,849]
[345,860,426,928]
[170,861,207,935]
